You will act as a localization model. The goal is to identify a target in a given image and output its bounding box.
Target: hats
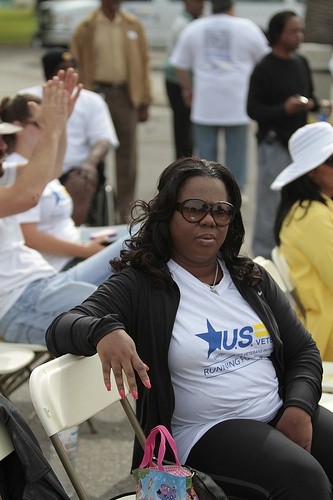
[0,120,23,135]
[271,121,333,190]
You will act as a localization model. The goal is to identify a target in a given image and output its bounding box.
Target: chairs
[0,339,271,500]
[253,245,333,413]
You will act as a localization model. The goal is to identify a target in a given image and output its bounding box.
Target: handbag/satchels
[132,425,199,500]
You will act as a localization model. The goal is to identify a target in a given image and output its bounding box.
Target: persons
[44,159,333,500]
[0,0,333,364]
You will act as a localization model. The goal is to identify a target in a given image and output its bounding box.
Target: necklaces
[204,260,220,296]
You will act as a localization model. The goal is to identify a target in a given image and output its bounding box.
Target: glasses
[174,198,236,227]
